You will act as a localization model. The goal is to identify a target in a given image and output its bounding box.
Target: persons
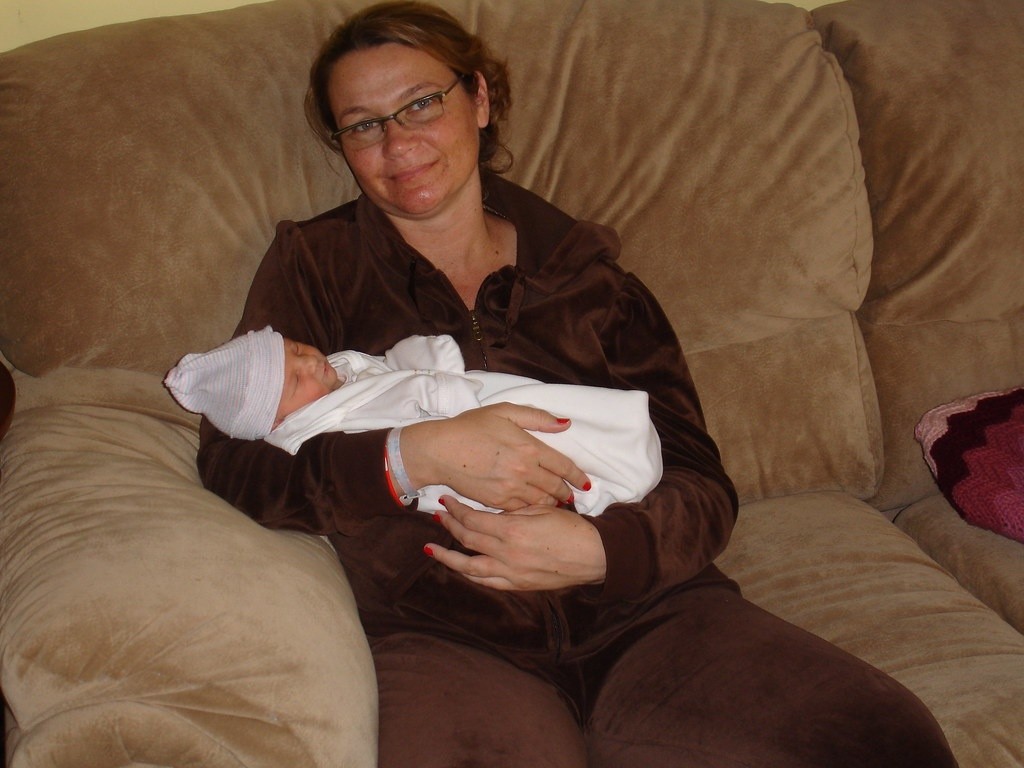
[165,326,665,519]
[196,0,961,768]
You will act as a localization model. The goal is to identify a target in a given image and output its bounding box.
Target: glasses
[330,74,467,152]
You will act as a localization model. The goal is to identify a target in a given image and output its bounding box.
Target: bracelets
[382,448,405,508]
[388,427,423,499]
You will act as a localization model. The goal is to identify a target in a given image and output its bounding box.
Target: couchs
[0,0,1024,768]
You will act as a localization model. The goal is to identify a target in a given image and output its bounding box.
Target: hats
[164,325,285,440]
